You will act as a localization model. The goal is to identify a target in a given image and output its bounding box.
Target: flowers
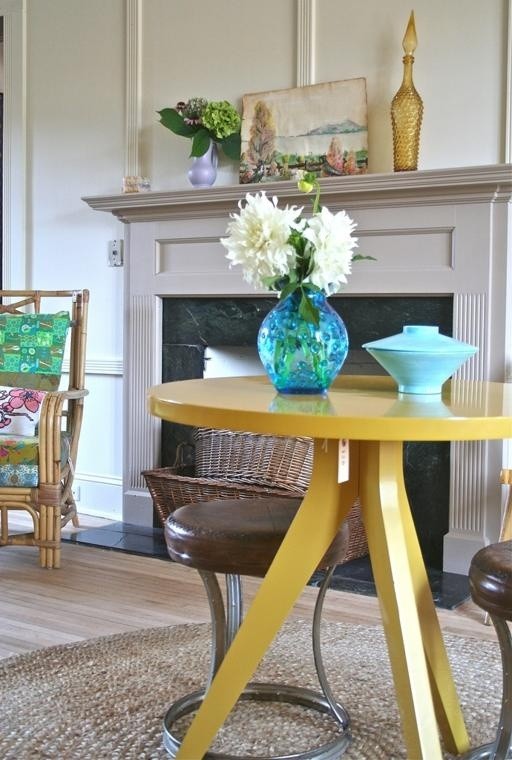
[222,172,358,320]
[155,101,240,157]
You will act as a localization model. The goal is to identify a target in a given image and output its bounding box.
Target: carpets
[0,622,504,760]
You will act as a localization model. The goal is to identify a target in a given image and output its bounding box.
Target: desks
[150,376,511,758]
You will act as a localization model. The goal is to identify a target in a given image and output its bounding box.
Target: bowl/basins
[362,349,479,395]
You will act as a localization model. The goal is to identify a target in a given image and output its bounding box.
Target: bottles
[390,10,426,171]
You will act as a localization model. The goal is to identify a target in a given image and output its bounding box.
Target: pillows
[2,310,70,391]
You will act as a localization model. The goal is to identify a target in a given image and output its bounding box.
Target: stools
[158,500,350,757]
[462,539,510,757]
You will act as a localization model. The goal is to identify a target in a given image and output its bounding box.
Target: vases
[187,134,221,188]
[255,284,351,395]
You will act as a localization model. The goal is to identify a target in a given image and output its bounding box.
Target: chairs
[0,288,90,572]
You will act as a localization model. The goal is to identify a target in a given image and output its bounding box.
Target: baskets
[140,426,370,569]
